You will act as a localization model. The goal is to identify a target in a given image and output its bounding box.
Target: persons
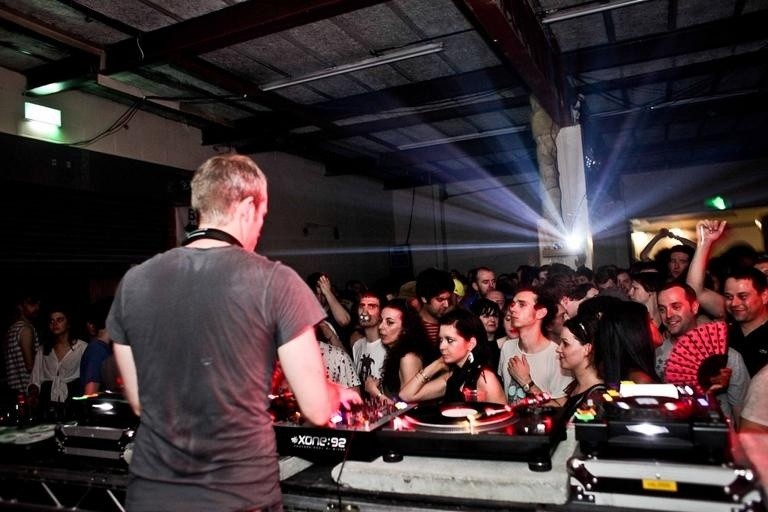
[739,365,768,495]
[79,298,115,394]
[84,311,99,337]
[26,308,88,413]
[106,155,363,512]
[303,219,768,422]
[7,289,41,406]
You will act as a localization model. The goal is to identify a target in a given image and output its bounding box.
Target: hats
[396,280,417,300]
[453,279,465,296]
